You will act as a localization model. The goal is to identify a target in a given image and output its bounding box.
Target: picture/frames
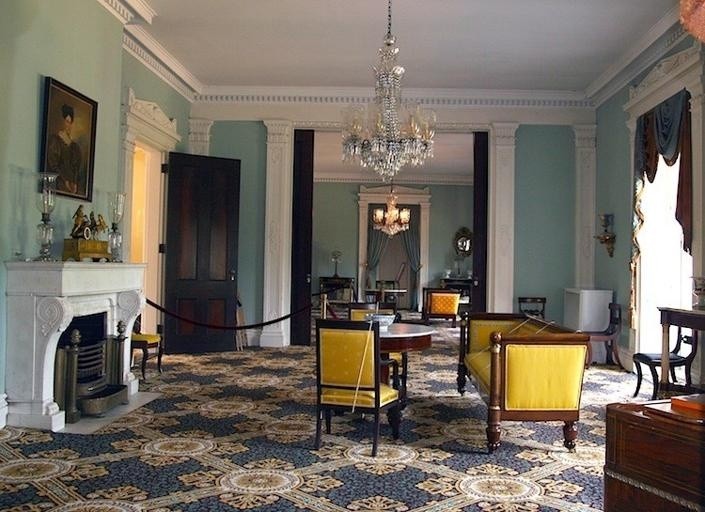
[39,75,99,204]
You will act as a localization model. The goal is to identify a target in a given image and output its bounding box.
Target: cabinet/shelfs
[604,394,705,512]
[318,276,355,307]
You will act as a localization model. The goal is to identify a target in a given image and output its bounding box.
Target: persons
[70,204,107,236]
[47,104,83,194]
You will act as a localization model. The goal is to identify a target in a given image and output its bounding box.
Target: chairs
[422,287,451,320]
[315,317,400,455]
[632,326,698,400]
[518,297,546,321]
[347,302,397,321]
[583,303,624,370]
[130,313,165,380]
[424,290,463,328]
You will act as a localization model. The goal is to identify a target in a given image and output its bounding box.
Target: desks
[364,288,407,302]
[379,320,433,410]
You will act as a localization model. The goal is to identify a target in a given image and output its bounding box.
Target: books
[642,393,705,426]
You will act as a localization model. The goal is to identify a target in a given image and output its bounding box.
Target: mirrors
[452,227,472,257]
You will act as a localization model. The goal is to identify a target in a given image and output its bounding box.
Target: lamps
[339,0,436,184]
[370,181,412,240]
[593,213,615,258]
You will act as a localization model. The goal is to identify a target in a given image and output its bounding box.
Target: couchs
[456,310,589,454]
